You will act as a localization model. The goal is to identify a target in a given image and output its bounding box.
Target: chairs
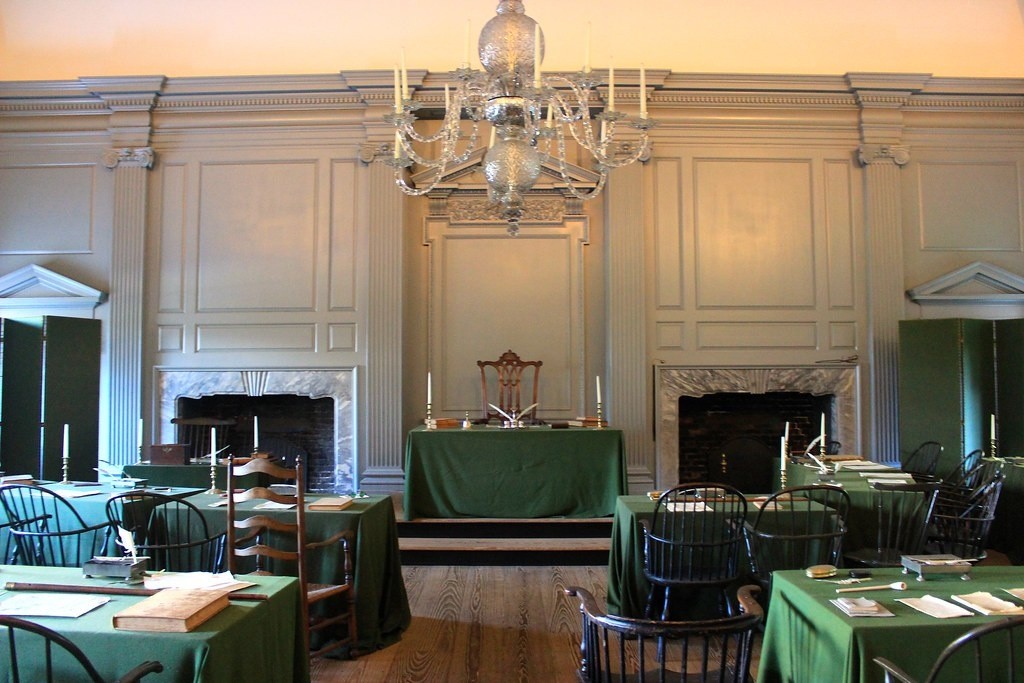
[171,417,237,459]
[819,440,1007,569]
[0,484,228,683]
[474,349,546,425]
[565,584,764,683]
[638,482,749,662]
[732,483,850,614]
[873,615,1024,683]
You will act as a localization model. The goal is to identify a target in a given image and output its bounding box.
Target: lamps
[857,143,910,166]
[102,146,155,169]
[379,0,663,238]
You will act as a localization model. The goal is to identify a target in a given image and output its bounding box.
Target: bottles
[463,411,471,429]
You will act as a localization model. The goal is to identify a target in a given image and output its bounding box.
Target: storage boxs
[150,443,192,465]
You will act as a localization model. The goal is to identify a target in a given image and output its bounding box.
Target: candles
[254,416,259,448]
[596,376,601,403]
[780,412,826,471]
[211,427,217,465]
[428,371,432,404]
[137,419,144,448]
[63,424,69,464]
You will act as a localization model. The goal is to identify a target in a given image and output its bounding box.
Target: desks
[0,458,411,683]
[226,453,360,673]
[774,453,927,549]
[401,422,628,519]
[605,494,839,639]
[976,457,1024,566]
[755,566,1024,683]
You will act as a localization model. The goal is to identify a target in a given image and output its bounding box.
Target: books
[567,416,608,426]
[1,475,33,484]
[838,597,878,614]
[951,592,1024,616]
[697,488,726,497]
[424,417,459,429]
[112,586,230,632]
[308,498,353,510]
[753,502,782,511]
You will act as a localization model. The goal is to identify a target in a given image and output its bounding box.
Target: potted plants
[991,414,995,445]
